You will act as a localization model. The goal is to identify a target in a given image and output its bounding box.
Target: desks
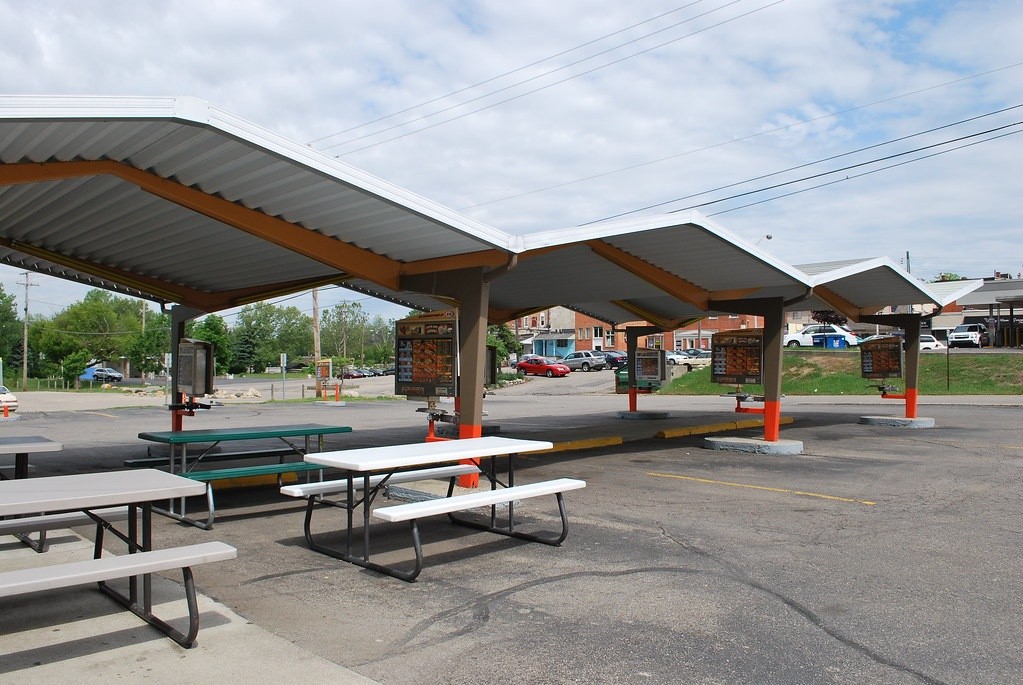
[303,436,554,583]
[0,468,206,640]
[0,435,65,481]
[137,422,353,529]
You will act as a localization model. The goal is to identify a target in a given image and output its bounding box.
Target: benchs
[372,477,586,583]
[280,464,482,547]
[0,540,238,646]
[0,463,36,480]
[124,447,331,529]
[0,505,142,553]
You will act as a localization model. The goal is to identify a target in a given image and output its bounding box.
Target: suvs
[556,349,607,372]
[947,323,988,349]
[93,367,123,382]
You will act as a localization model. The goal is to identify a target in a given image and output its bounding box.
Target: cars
[337,370,364,380]
[370,363,396,377]
[602,349,627,370]
[0,386,18,413]
[920,332,946,351]
[783,323,864,349]
[509,354,537,369]
[356,370,374,378]
[665,347,713,372]
[515,357,571,378]
[863,335,905,351]
